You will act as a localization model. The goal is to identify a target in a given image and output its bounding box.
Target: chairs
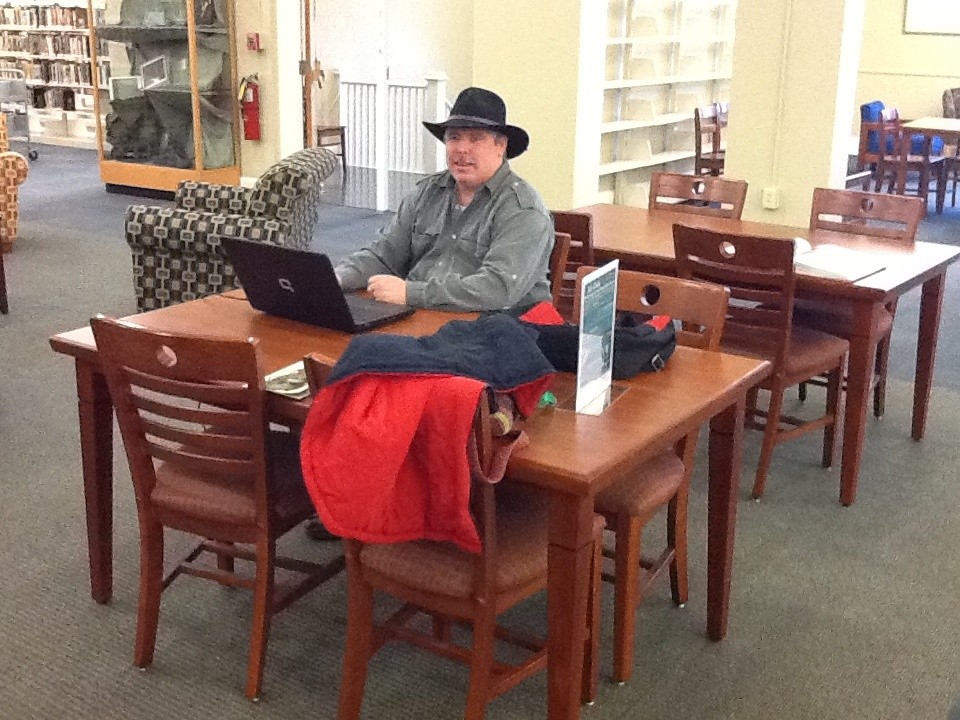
[87,88,960,719]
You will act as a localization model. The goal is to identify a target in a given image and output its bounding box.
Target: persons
[302,87,556,543]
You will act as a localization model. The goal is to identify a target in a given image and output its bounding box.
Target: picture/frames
[902,0,960,35]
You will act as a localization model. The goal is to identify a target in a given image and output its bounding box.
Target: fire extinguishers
[238,75,262,140]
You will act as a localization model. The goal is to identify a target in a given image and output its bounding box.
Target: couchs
[125,148,336,316]
[0,111,29,251]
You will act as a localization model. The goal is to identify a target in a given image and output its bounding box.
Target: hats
[423,87,529,159]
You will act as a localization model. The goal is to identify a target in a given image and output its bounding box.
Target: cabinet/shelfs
[0,0,116,153]
[90,0,241,200]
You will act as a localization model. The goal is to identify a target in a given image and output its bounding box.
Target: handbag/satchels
[479,302,676,380]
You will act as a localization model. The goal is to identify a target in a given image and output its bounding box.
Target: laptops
[220,237,416,335]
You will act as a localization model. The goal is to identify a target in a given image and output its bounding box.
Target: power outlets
[761,185,782,211]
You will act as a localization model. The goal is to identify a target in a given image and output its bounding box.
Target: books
[0,1,110,110]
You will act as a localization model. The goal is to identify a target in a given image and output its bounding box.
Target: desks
[49,281,764,720]
[897,116,960,212]
[535,203,960,507]
